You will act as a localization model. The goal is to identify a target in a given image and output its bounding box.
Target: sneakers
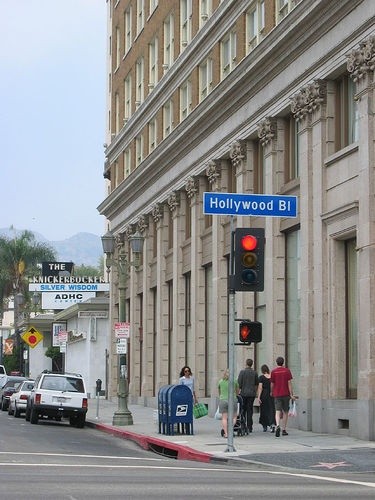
[275,426,280,437]
[282,430,288,435]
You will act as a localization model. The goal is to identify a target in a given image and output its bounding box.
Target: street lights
[102,222,142,426]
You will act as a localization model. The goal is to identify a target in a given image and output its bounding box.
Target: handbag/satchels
[193,401,208,418]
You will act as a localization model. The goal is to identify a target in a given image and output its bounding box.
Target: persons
[237,358,259,432]
[217,368,240,438]
[257,364,275,432]
[270,357,298,437]
[178,366,199,434]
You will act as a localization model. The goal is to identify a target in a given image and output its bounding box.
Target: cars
[0,366,5,377]
[26,369,87,427]
[0,377,27,410]
[9,381,33,417]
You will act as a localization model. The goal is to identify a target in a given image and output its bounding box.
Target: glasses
[184,370,190,373]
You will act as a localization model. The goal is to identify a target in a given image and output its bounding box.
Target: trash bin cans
[162,384,184,435]
[158,384,174,434]
[168,384,193,435]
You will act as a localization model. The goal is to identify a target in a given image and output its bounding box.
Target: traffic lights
[231,229,264,291]
[239,322,262,342]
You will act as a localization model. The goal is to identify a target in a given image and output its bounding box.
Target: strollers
[223,394,249,435]
